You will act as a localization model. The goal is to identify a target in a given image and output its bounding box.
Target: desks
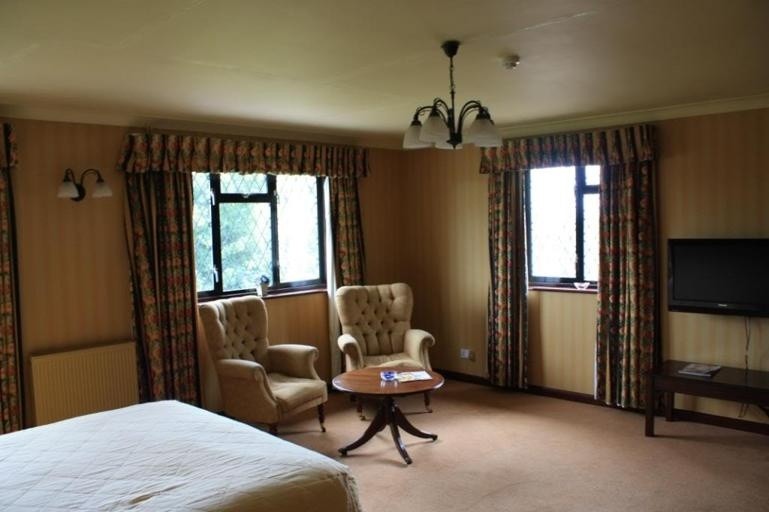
[330,368,446,467]
[641,359,769,438]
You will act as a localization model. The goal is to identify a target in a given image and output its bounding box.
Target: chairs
[197,295,329,438]
[336,282,436,419]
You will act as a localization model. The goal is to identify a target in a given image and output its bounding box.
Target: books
[678,363,721,377]
[396,369,434,383]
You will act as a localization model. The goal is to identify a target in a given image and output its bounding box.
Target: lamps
[57,167,114,202]
[400,40,505,153]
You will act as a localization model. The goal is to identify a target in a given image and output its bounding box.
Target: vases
[256,285,268,296]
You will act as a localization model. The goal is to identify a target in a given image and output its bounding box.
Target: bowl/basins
[574,281,591,290]
[381,370,398,381]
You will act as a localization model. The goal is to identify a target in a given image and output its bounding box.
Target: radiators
[27,341,143,427]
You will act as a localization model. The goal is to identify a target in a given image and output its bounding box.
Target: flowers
[253,274,270,287]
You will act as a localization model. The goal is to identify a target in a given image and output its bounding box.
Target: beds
[1,398,361,512]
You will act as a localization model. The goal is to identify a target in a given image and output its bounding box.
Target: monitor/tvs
[667,238,768,319]
